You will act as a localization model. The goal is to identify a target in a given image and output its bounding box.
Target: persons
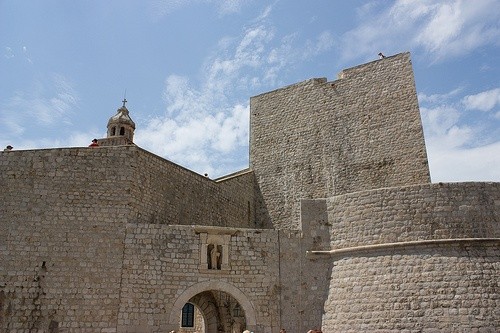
[6,145,13,152]
[377,52,386,59]
[88,139,100,148]
[204,173,210,179]
